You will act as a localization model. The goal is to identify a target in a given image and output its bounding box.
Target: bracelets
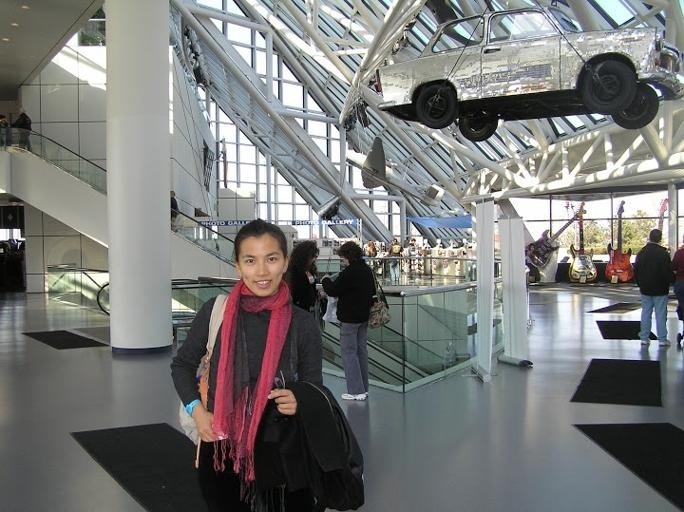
[185,399,201,416]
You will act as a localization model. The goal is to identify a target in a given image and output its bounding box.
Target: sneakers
[341,391,365,400]
[640,339,651,345]
[658,339,671,345]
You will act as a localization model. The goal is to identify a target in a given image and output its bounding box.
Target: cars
[373,6,684,143]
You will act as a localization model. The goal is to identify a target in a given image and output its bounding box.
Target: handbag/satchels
[368,302,390,329]
[179,353,209,446]
[378,292,390,308]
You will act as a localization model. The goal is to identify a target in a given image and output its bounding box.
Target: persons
[170,190,179,231]
[283,240,328,329]
[365,238,416,281]
[633,228,676,346]
[671,234,684,321]
[168,219,325,512]
[321,241,378,401]
[10,108,32,150]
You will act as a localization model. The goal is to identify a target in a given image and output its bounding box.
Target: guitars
[569,203,597,283]
[525,209,587,267]
[605,202,634,283]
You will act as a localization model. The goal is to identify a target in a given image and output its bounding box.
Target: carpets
[529,279,660,343]
[567,358,665,409]
[22,330,110,350]
[68,423,209,511]
[570,421,684,512]
